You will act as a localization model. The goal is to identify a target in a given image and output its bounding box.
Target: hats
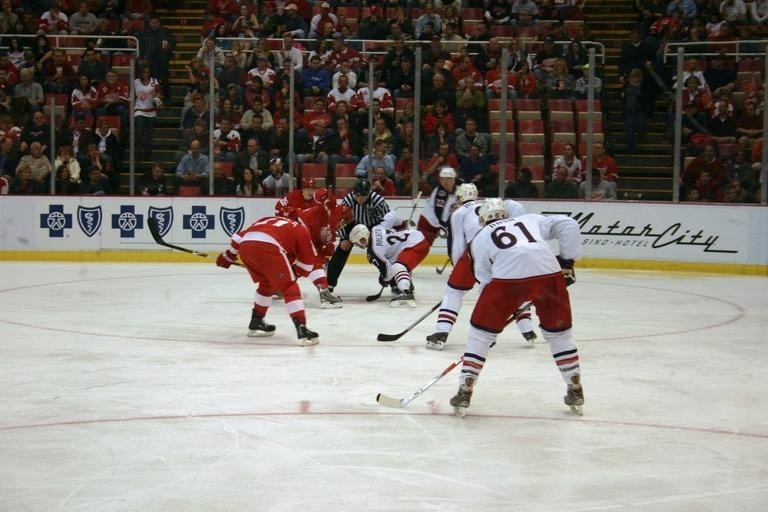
[192,94,204,102]
[283,3,298,12]
[321,2,330,8]
[199,71,209,79]
[227,83,240,93]
[367,54,378,60]
[544,36,552,42]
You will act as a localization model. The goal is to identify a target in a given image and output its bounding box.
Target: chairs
[103,74,130,88]
[96,115,121,135]
[61,37,85,47]
[396,98,414,109]
[69,113,94,129]
[539,20,560,41]
[564,21,583,38]
[107,20,119,33]
[43,113,61,130]
[301,2,485,39]
[301,164,327,190]
[95,54,111,68]
[486,96,604,196]
[213,161,236,178]
[489,23,512,38]
[307,97,326,109]
[523,41,543,58]
[46,93,68,108]
[112,53,134,66]
[672,57,766,204]
[130,19,146,33]
[60,54,82,66]
[334,163,362,196]
[178,185,202,197]
[515,23,538,41]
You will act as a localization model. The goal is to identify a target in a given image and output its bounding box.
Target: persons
[274,177,318,219]
[216,205,319,341]
[175,0,617,200]
[348,210,430,304]
[1,1,179,199]
[273,187,354,303]
[449,197,585,407]
[327,180,390,291]
[425,182,538,345]
[417,165,463,257]
[617,1,768,202]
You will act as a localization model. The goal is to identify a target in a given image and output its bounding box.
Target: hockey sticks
[147,217,249,268]
[378,301,442,340]
[366,190,423,301]
[376,275,576,409]
[435,258,452,274]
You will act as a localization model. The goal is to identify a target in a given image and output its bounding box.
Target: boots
[450,385,474,408]
[522,331,538,339]
[564,384,583,405]
[316,284,343,303]
[249,309,276,331]
[390,291,415,299]
[292,317,319,339]
[426,332,448,343]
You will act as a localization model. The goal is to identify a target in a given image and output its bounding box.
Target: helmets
[277,206,298,221]
[351,179,371,195]
[333,205,355,231]
[439,167,458,178]
[454,182,479,205]
[478,198,509,227]
[349,223,370,249]
[301,177,316,189]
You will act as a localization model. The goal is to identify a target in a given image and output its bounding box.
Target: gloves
[315,187,328,203]
[379,274,389,287]
[556,255,576,287]
[216,249,237,269]
